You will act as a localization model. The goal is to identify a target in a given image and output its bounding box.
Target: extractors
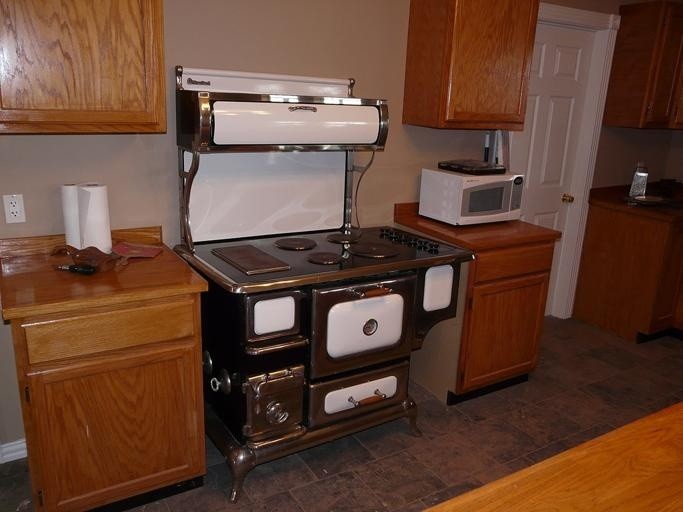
[176,65,389,154]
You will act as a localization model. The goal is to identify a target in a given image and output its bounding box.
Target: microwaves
[417,168,526,226]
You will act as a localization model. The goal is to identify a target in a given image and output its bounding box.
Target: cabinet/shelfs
[0,1,170,134]
[393,200,561,407]
[604,2,683,130]
[0,226,208,511]
[402,0,540,132]
[572,181,683,343]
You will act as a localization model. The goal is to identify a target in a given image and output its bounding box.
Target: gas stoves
[217,225,408,278]
